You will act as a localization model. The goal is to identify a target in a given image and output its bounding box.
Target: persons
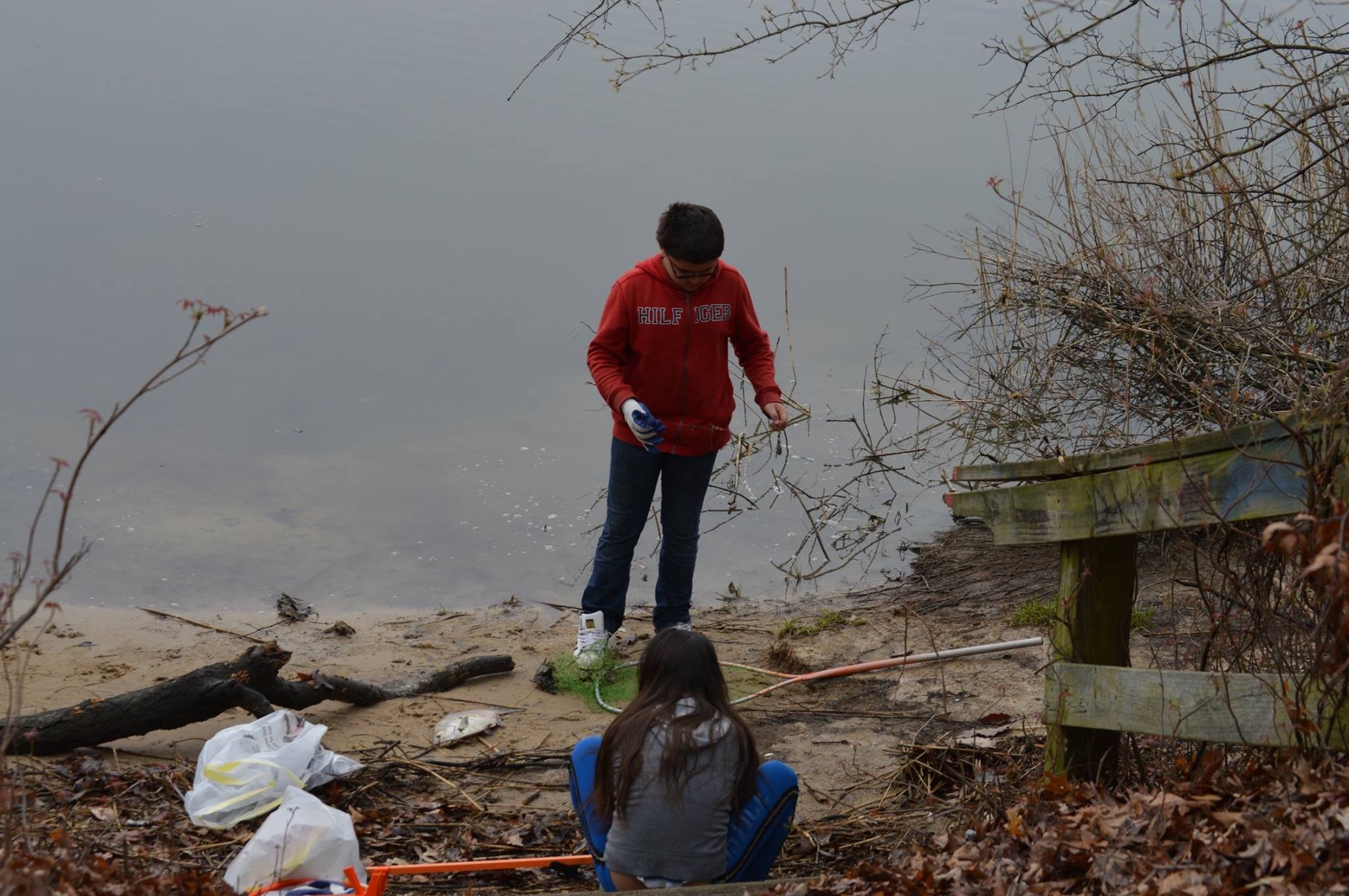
[567,625,800,893]
[573,201,790,669]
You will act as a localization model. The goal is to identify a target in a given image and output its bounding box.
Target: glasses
[665,254,718,279]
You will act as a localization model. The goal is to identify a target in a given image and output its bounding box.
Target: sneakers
[572,610,609,671]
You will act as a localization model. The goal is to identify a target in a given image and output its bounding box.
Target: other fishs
[432,708,523,747]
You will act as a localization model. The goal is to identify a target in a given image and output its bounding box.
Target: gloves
[621,399,667,453]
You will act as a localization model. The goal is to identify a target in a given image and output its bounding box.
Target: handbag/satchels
[183,709,367,830]
[220,786,368,896]
